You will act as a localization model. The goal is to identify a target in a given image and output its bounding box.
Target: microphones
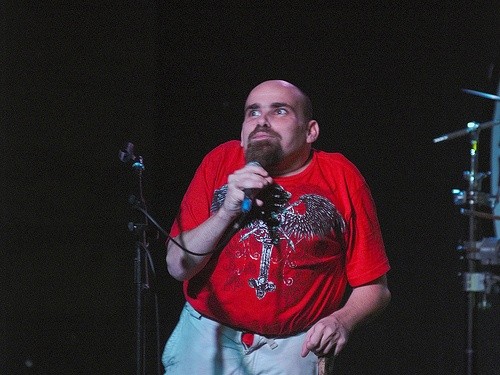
[241,161,262,213]
